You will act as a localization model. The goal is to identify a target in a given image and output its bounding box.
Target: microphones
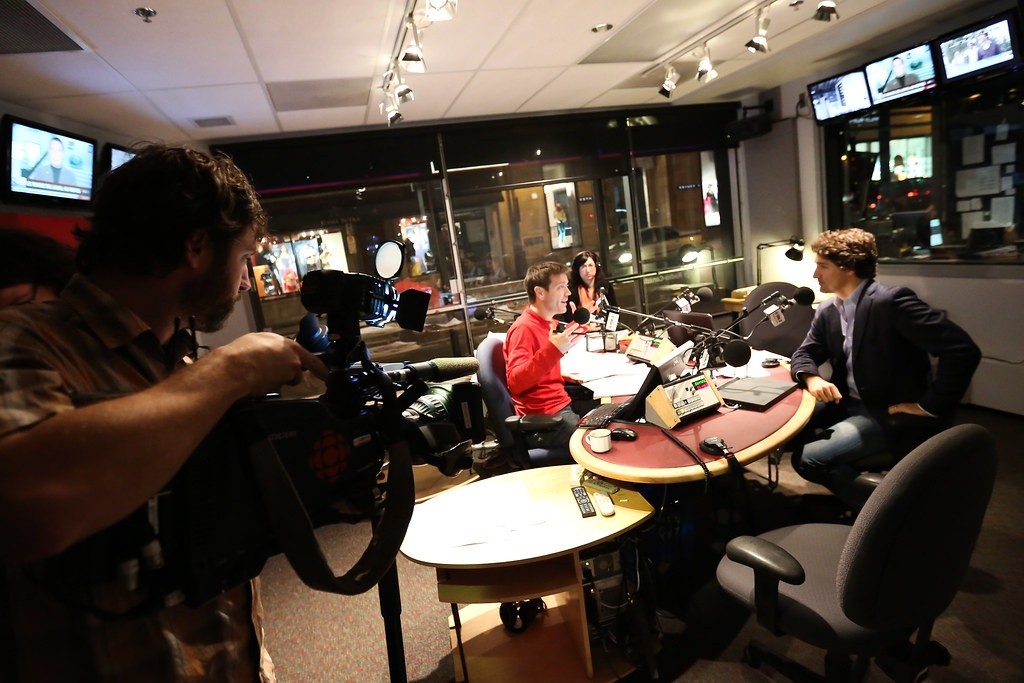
[474,308,506,326]
[763,286,815,328]
[385,357,480,383]
[697,287,713,303]
[723,339,751,367]
[573,308,619,332]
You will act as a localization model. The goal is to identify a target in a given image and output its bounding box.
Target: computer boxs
[577,542,627,641]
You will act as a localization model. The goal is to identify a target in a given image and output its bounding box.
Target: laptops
[662,311,717,360]
[717,377,799,412]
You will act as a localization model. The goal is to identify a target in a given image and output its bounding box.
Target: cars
[608,226,708,276]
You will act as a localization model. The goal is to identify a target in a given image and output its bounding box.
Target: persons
[791,229,982,508]
[964,33,1002,63]
[485,252,507,284]
[553,204,567,248]
[33,138,75,183]
[563,251,617,322]
[502,261,598,464]
[887,58,920,92]
[463,253,484,288]
[706,184,718,211]
[0,146,331,683]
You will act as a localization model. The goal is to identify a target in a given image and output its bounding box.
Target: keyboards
[578,402,624,429]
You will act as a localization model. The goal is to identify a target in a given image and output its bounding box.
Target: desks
[398,297,816,682]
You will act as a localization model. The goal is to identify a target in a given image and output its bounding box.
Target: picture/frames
[543,181,583,251]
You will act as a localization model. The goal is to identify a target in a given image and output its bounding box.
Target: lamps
[377,0,460,128]
[756,237,807,287]
[745,29,767,53]
[812,1,840,23]
[658,72,680,98]
[679,244,720,294]
[696,56,718,82]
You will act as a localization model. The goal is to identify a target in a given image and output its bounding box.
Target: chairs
[716,282,996,683]
[478,334,586,470]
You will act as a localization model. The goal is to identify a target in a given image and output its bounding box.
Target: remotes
[571,478,620,518]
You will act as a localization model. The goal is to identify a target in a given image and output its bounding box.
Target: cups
[585,429,612,453]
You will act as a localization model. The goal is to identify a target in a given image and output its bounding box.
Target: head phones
[700,437,749,506]
[500,599,539,634]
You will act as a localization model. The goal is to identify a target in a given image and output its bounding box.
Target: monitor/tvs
[2,113,98,209]
[102,142,138,172]
[620,341,694,421]
[806,7,1024,127]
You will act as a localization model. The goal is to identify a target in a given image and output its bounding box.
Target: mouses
[610,428,637,441]
